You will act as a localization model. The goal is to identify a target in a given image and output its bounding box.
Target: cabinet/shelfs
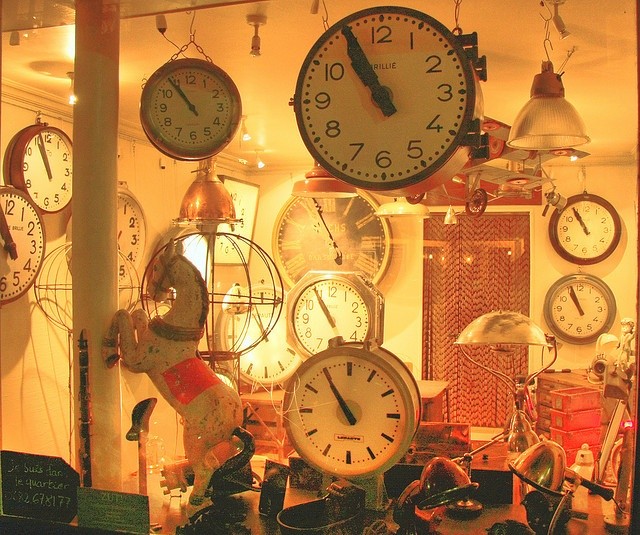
[535,366,618,457]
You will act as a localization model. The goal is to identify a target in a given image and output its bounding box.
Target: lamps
[173,156,241,227]
[505,1,592,151]
[452,314,560,455]
[294,167,348,192]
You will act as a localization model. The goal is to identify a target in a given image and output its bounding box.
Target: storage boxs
[549,386,602,413]
[565,445,603,468]
[549,426,603,450]
[549,408,604,432]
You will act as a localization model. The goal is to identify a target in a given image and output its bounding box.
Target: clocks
[12,125,72,214]
[67,181,149,283]
[548,188,623,267]
[270,191,392,292]
[544,270,617,346]
[288,7,488,197]
[289,272,384,357]
[282,337,422,480]
[1,186,44,307]
[140,60,241,160]
[218,281,307,394]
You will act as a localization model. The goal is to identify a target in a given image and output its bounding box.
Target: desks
[417,380,452,427]
[239,390,293,459]
